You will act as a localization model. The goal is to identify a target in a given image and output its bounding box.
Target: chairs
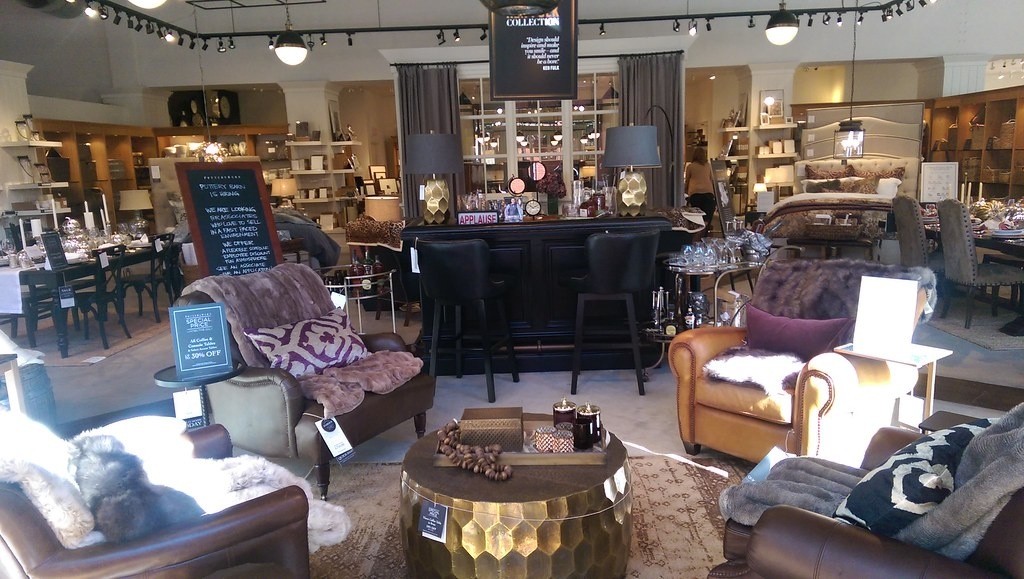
[891,195,954,317]
[0,222,184,358]
[980,252,1024,318]
[937,199,1024,328]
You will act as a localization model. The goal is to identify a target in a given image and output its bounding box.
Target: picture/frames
[363,164,399,197]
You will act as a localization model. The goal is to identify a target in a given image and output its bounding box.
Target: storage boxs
[460,407,526,451]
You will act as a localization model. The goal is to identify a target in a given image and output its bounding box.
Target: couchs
[1,407,308,579]
[152,263,431,501]
[703,402,1024,579]
[666,250,938,462]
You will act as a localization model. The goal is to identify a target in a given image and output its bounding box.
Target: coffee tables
[400,411,632,579]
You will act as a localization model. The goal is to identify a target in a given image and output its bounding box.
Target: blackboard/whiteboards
[174,161,284,279]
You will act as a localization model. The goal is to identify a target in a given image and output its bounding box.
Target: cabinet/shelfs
[922,86,1024,202]
[286,140,360,202]
[754,123,798,187]
[717,125,748,160]
[153,126,293,181]
[6,137,72,216]
[31,117,156,228]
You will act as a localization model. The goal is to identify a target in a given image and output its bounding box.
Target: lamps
[67,0,937,66]
[404,132,464,222]
[119,189,153,239]
[600,125,662,215]
[834,1,865,159]
[478,101,600,147]
[764,168,794,203]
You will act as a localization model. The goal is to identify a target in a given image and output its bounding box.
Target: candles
[52,199,58,228]
[966,181,972,207]
[978,181,983,199]
[84,201,89,212]
[100,210,107,228]
[20,219,27,250]
[102,194,110,225]
[960,183,964,204]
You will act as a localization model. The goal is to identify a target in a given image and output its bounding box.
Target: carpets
[0,296,174,368]
[925,282,1024,351]
[219,435,746,578]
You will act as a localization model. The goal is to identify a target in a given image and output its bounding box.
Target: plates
[127,243,151,248]
[44,253,82,264]
[922,217,938,224]
[992,230,1024,239]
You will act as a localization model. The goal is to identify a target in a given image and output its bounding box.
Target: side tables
[918,410,984,436]
[834,341,952,434]
[156,363,242,427]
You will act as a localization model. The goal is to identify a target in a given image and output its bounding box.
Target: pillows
[244,307,368,385]
[742,300,850,361]
[800,164,905,198]
[831,417,990,536]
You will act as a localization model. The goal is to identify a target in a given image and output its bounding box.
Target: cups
[573,417,594,449]
[535,426,556,453]
[552,431,574,454]
[1006,199,1015,208]
[576,401,602,442]
[557,422,573,431]
[552,397,577,427]
[926,204,936,214]
[603,186,618,218]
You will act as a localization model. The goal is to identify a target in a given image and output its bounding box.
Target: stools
[569,227,665,397]
[419,242,520,402]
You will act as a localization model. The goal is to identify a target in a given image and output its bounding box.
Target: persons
[684,146,717,230]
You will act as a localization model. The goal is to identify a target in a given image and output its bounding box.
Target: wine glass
[1,218,150,269]
[669,235,739,271]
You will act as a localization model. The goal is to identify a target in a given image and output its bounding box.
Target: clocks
[526,199,540,216]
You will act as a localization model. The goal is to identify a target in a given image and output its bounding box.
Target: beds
[761,157,920,240]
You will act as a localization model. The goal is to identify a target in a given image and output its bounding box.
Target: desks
[915,208,1024,335]
[0,238,181,360]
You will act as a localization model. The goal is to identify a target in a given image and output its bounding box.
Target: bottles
[322,246,385,296]
[650,286,743,334]
[593,191,605,211]
[580,197,597,217]
